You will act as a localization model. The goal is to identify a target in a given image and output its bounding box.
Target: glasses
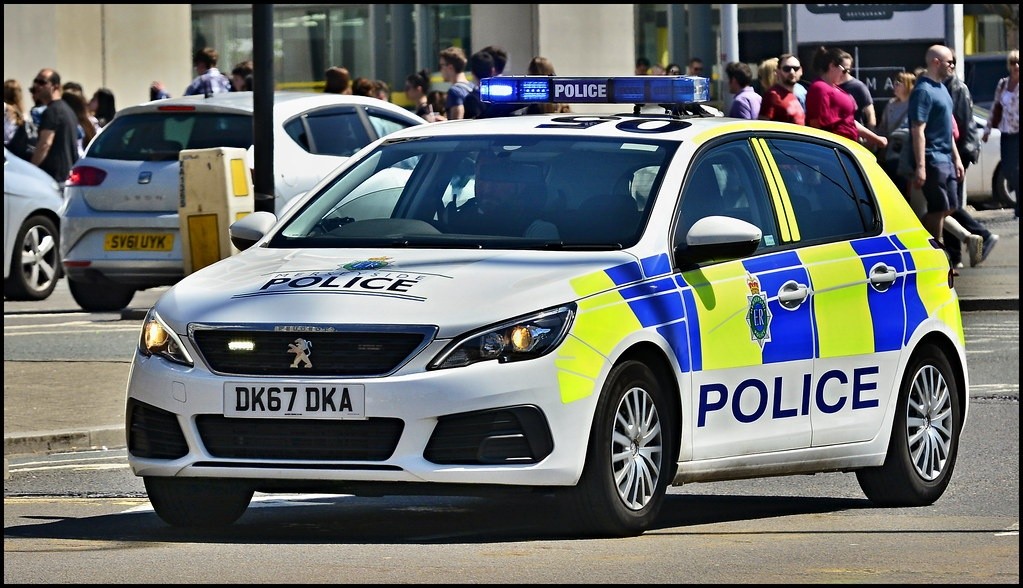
[894,80,902,86]
[33,78,49,86]
[945,60,956,66]
[404,85,417,92]
[838,65,851,75]
[1009,59,1019,66]
[437,62,450,70]
[692,66,703,71]
[781,66,800,72]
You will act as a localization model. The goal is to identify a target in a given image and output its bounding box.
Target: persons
[4,68,116,183]
[758,57,807,110]
[232,60,253,91]
[453,148,527,236]
[462,46,507,120]
[982,49,1019,216]
[323,66,389,100]
[725,61,763,120]
[805,46,888,147]
[152,47,230,99]
[524,56,570,115]
[835,72,876,153]
[436,47,475,122]
[757,54,806,126]
[636,57,703,76]
[877,72,916,138]
[405,68,434,122]
[907,45,1000,268]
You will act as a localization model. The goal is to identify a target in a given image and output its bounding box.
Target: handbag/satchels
[991,103,1003,128]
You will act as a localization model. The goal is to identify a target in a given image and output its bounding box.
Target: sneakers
[982,234,998,261]
[965,234,982,267]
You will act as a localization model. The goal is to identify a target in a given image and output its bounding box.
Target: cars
[913,113,1015,211]
[58,88,477,315]
[4,149,63,302]
[120,73,968,535]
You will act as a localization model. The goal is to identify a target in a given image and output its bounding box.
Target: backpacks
[5,109,38,162]
